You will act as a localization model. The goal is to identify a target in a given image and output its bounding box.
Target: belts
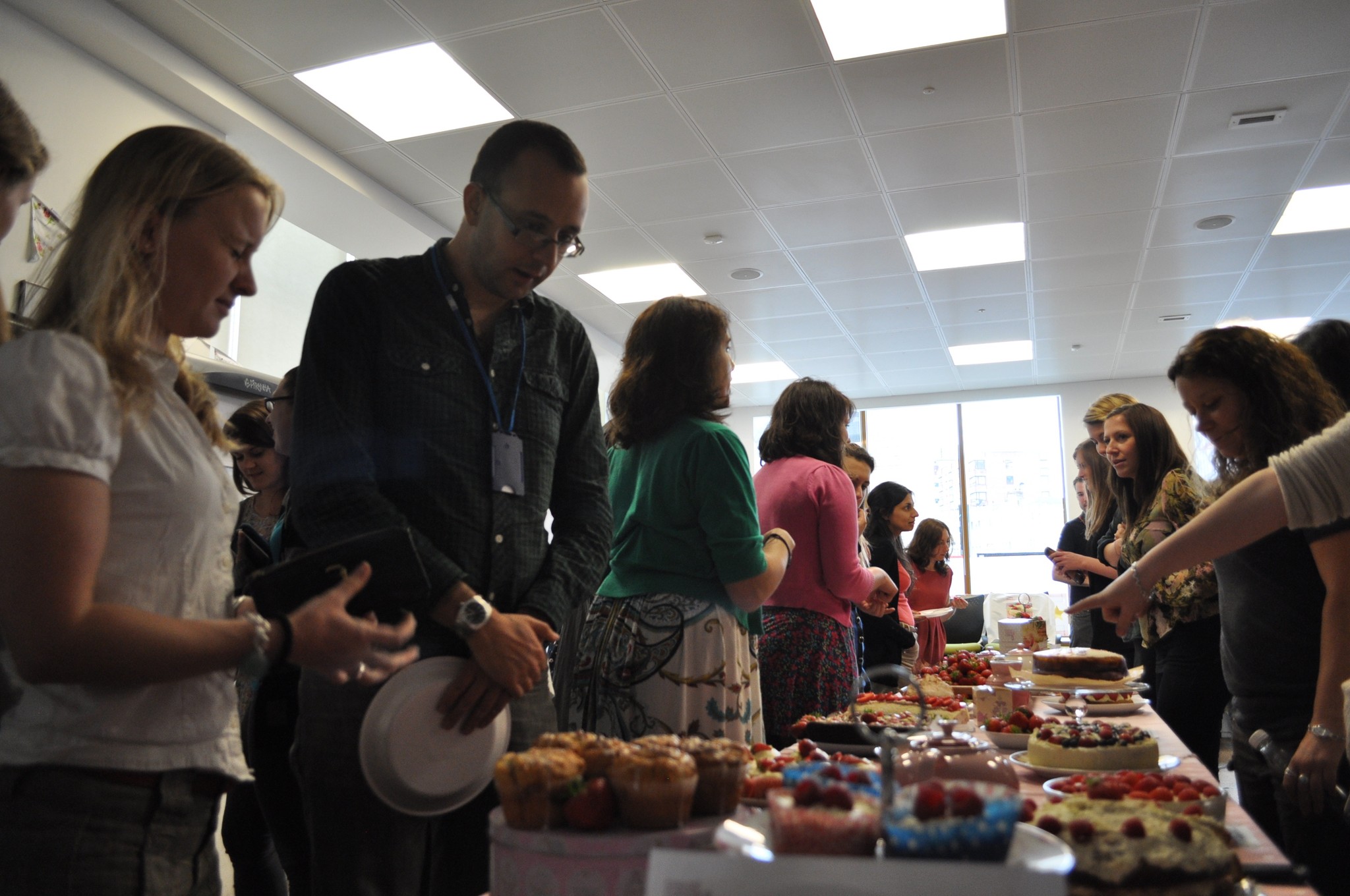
[900,623,917,633]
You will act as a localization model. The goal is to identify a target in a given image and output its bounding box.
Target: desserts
[740,737,1024,864]
[493,729,753,835]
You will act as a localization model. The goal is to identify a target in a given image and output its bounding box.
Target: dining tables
[781,662,1316,896]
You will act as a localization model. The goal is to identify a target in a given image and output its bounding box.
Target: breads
[904,673,954,697]
[971,685,1013,726]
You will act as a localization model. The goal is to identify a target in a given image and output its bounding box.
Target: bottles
[975,646,1001,662]
[985,661,1022,686]
[1248,729,1349,816]
[1004,642,1035,670]
[1045,547,1085,585]
[891,718,1019,790]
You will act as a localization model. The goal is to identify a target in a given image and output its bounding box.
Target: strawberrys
[919,648,992,685]
[985,705,1060,734]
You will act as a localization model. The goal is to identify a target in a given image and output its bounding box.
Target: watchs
[456,595,493,636]
[764,533,792,567]
[1307,724,1345,743]
[233,595,253,610]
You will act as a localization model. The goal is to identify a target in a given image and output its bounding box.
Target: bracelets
[276,615,293,657]
[1131,561,1147,597]
[244,613,270,662]
[1114,541,1120,556]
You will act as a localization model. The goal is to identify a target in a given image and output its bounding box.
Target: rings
[355,662,366,679]
[1298,774,1308,784]
[1283,767,1297,781]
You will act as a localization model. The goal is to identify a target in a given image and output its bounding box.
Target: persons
[1082,395,1152,711]
[293,121,612,895]
[744,378,897,749]
[0,78,968,896]
[0,124,418,896]
[1048,316,1350,671]
[544,298,797,742]
[1168,327,1350,896]
[1102,403,1235,782]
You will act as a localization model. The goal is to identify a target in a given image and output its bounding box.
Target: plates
[360,656,511,815]
[912,607,953,618]
[805,721,915,745]
[795,739,880,758]
[949,685,971,695]
[1044,776,1230,820]
[713,805,1075,876]
[1008,749,1182,780]
[1040,696,1153,715]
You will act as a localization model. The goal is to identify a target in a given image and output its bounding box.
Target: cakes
[997,613,1048,652]
[1017,795,1239,896]
[1061,692,1135,705]
[1027,720,1160,770]
[1052,773,1226,825]
[792,691,971,744]
[1031,647,1129,682]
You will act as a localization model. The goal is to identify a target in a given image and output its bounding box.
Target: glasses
[264,395,293,412]
[484,182,585,259]
